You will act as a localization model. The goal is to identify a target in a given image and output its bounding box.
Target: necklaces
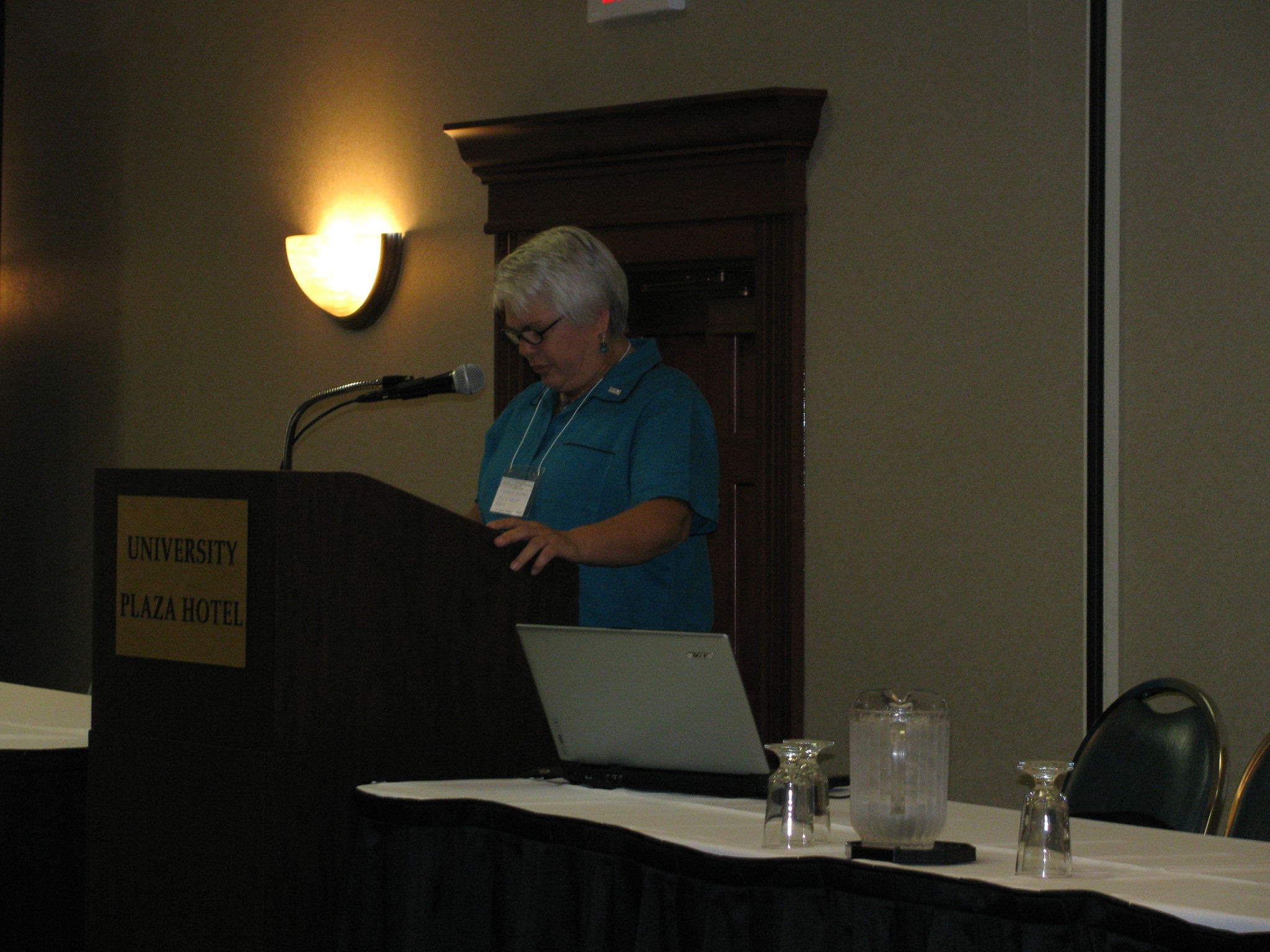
[560,377,601,412]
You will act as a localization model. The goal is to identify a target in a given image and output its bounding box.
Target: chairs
[1220,744,1269,846]
[1060,679,1225,841]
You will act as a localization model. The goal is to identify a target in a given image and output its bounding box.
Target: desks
[355,768,1270,952]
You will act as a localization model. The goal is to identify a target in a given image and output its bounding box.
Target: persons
[465,225,719,634]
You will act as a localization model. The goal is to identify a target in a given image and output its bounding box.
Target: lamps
[284,223,382,319]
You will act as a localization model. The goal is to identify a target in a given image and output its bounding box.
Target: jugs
[850,688,951,851]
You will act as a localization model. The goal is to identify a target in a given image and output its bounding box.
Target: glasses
[501,311,570,345]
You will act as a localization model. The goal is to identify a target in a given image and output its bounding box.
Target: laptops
[516,624,850,799]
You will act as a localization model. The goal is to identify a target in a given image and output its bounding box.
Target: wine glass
[1016,761,1074,878]
[763,744,814,849]
[783,739,834,843]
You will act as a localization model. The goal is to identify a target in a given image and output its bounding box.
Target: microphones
[354,363,485,402]
